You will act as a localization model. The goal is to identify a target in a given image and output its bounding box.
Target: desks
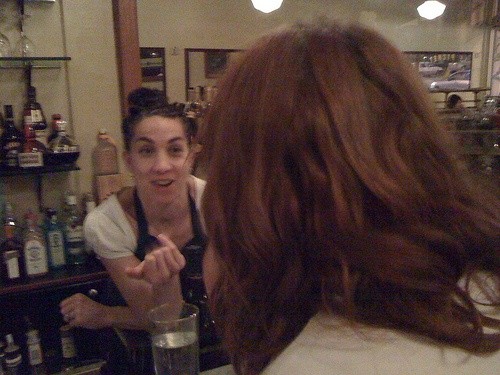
[0,254,109,339]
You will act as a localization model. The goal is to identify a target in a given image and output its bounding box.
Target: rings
[68,312,70,319]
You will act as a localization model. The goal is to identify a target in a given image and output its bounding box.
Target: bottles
[1,86,79,172]
[1,193,100,282]
[188,84,217,103]
[1,314,79,375]
[91,129,126,201]
[424,53,457,67]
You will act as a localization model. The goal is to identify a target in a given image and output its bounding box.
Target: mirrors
[139,46,166,97]
[184,48,249,102]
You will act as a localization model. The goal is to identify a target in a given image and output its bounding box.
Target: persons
[60,86,206,340]
[199,21,500,375]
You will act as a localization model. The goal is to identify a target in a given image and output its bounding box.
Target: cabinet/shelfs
[0,56,82,177]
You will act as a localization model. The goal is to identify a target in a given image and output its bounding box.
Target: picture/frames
[205,51,231,78]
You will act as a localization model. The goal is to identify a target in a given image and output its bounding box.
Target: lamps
[251,0,283,14]
[416,0,446,20]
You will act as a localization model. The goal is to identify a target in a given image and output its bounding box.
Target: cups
[150,302,200,375]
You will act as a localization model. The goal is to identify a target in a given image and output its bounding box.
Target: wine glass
[1,10,35,57]
